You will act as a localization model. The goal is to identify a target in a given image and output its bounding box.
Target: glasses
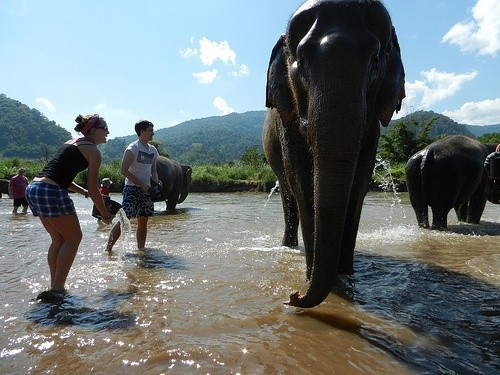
[94,125,108,131]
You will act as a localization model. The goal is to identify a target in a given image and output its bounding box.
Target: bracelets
[85,190,88,198]
[102,215,112,220]
[81,188,84,194]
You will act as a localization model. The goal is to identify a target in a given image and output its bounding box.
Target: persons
[97,178,113,224]
[25,114,113,297]
[8,168,29,213]
[104,120,163,252]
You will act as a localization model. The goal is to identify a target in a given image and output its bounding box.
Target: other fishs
[105,221,121,254]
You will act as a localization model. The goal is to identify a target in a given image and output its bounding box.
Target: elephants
[261,0,406,309]
[150,156,192,213]
[404,135,500,231]
[0,179,12,199]
